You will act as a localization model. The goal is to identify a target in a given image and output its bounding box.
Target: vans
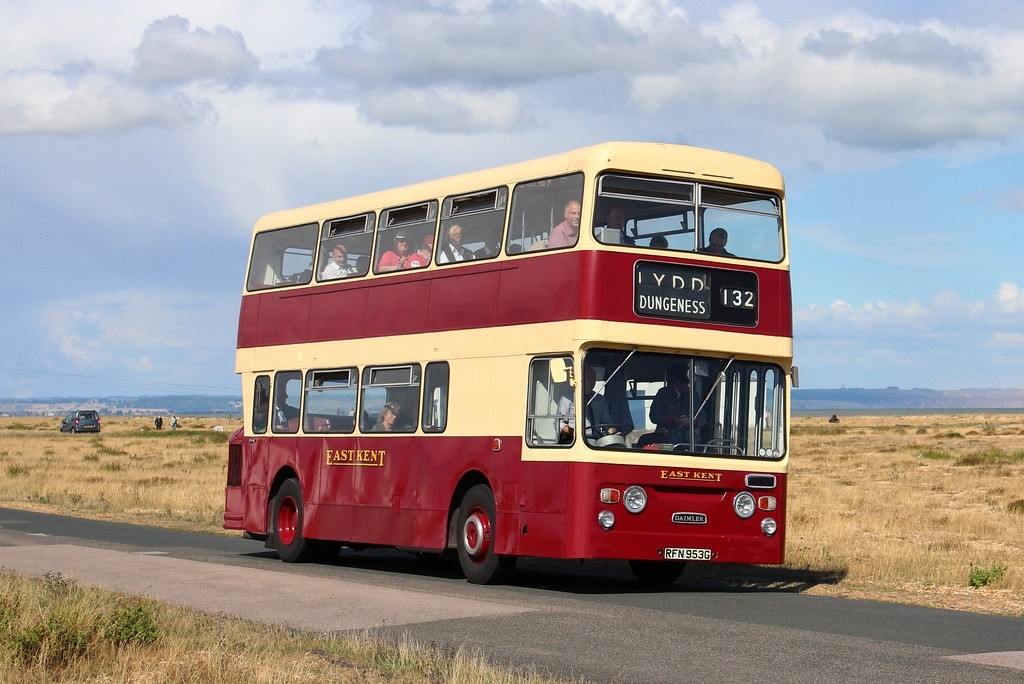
[59,410,100,435]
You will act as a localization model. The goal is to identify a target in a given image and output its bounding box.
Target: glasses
[397,237,410,243]
[711,242,725,248]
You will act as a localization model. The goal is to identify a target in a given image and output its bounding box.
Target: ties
[585,393,596,434]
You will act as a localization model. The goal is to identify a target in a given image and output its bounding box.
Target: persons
[155,415,163,431]
[596,205,636,246]
[439,224,474,263]
[649,233,669,250]
[691,227,736,257]
[168,414,178,431]
[547,199,582,249]
[297,244,330,284]
[336,406,369,433]
[320,244,358,280]
[377,229,411,272]
[368,401,431,432]
[402,234,434,268]
[473,228,501,259]
[648,366,708,452]
[556,367,618,438]
[259,390,289,427]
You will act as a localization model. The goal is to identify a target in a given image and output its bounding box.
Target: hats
[672,365,691,383]
[395,230,410,239]
[422,234,433,244]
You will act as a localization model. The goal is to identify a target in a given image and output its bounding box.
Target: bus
[222,140,800,584]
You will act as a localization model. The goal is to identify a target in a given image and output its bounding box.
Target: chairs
[282,408,380,432]
[282,251,363,284]
